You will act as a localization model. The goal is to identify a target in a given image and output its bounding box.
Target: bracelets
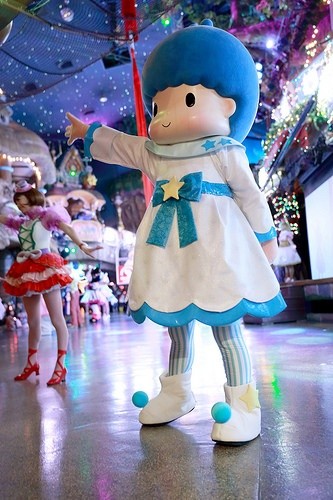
[79,243,83,248]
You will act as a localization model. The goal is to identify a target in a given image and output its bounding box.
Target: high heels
[13,348,39,381]
[47,349,66,385]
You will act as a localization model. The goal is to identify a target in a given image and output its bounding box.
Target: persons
[64,16,288,442]
[0,179,103,386]
[272,216,302,283]
